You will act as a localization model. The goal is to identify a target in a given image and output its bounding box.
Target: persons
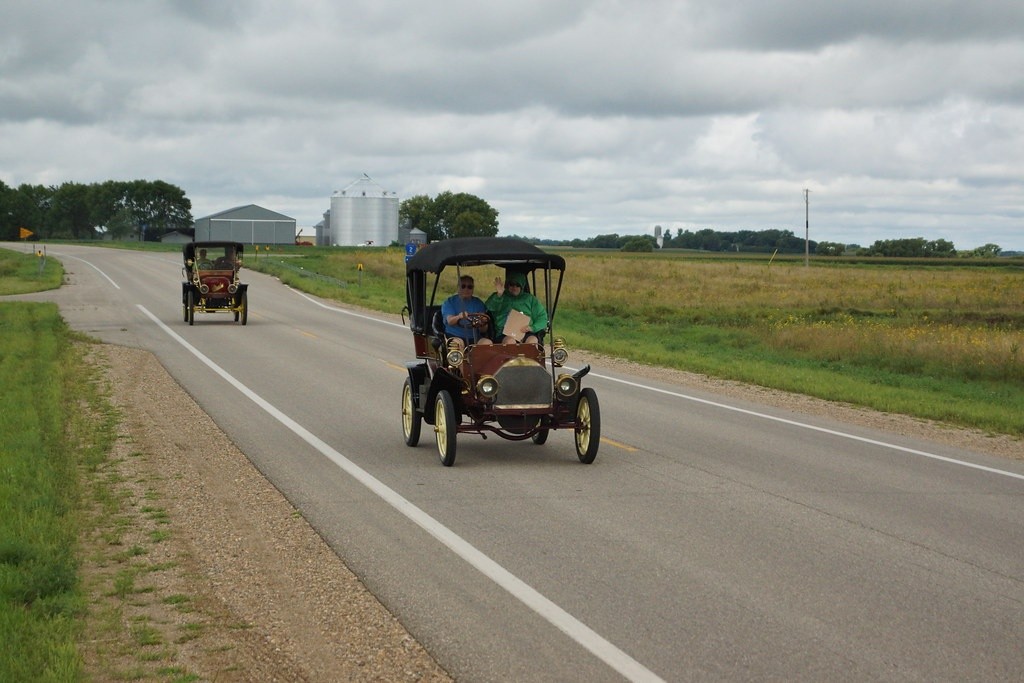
[193,249,214,275]
[484,272,547,351]
[442,275,493,356]
[216,248,239,272]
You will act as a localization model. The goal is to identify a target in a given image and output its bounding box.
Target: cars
[179,240,250,325]
[400,231,602,467]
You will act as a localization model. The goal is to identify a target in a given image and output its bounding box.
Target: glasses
[509,281,521,287]
[459,284,474,290]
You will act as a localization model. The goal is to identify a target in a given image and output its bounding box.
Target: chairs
[432,311,494,356]
